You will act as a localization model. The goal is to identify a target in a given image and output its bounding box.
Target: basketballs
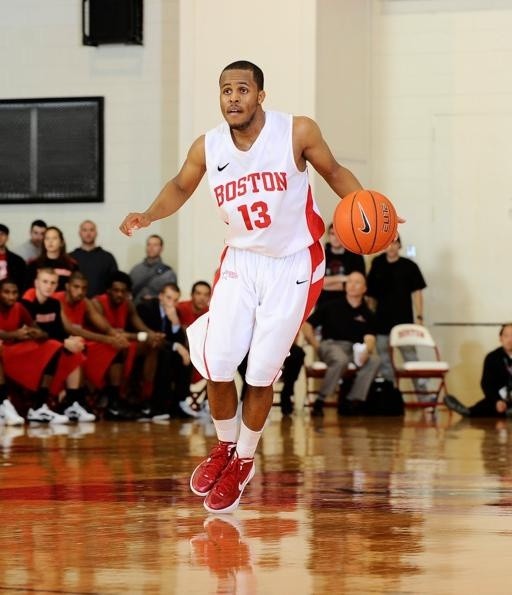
[335,188,398,256]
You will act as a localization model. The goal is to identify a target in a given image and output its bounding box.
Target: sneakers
[281,394,293,415]
[103,409,136,422]
[137,409,170,422]
[190,441,235,497]
[202,451,256,512]
[27,403,68,423]
[0,399,24,425]
[311,401,324,419]
[64,401,96,422]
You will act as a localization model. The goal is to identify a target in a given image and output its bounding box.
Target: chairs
[303,329,358,414]
[268,366,295,407]
[389,323,453,415]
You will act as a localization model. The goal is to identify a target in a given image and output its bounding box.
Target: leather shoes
[443,394,471,417]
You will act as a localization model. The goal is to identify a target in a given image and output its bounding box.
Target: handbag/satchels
[337,375,406,417]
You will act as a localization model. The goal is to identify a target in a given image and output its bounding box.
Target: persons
[0,279,64,427]
[25,227,79,292]
[314,223,366,324]
[67,220,119,298]
[443,323,512,419]
[53,271,136,422]
[0,224,28,295]
[176,280,215,418]
[302,272,381,418]
[127,282,192,420]
[126,234,180,307]
[89,278,170,421]
[366,236,438,412]
[238,332,305,417]
[12,220,48,263]
[17,266,97,423]
[118,61,406,513]
